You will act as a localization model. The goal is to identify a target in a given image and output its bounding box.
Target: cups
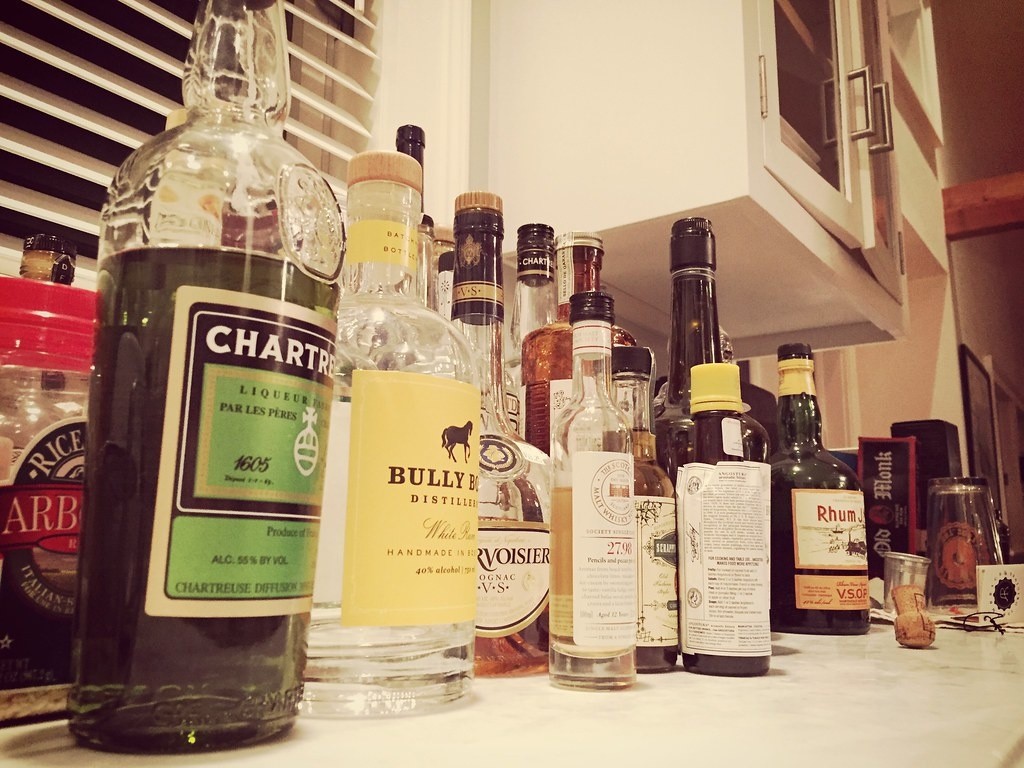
[883,551,932,615]
[927,476,1004,614]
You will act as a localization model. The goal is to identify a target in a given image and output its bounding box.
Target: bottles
[395,125,438,308]
[296,151,480,720]
[548,290,638,691]
[770,343,870,637]
[505,224,558,442]
[612,347,679,672]
[653,217,769,471]
[448,190,548,678]
[437,251,455,320]
[521,231,637,456]
[0,235,94,728]
[668,364,771,677]
[66,0,347,755]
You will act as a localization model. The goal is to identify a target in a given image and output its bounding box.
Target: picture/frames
[959,342,1004,514]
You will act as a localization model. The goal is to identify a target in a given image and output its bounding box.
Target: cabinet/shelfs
[487,0,908,360]
